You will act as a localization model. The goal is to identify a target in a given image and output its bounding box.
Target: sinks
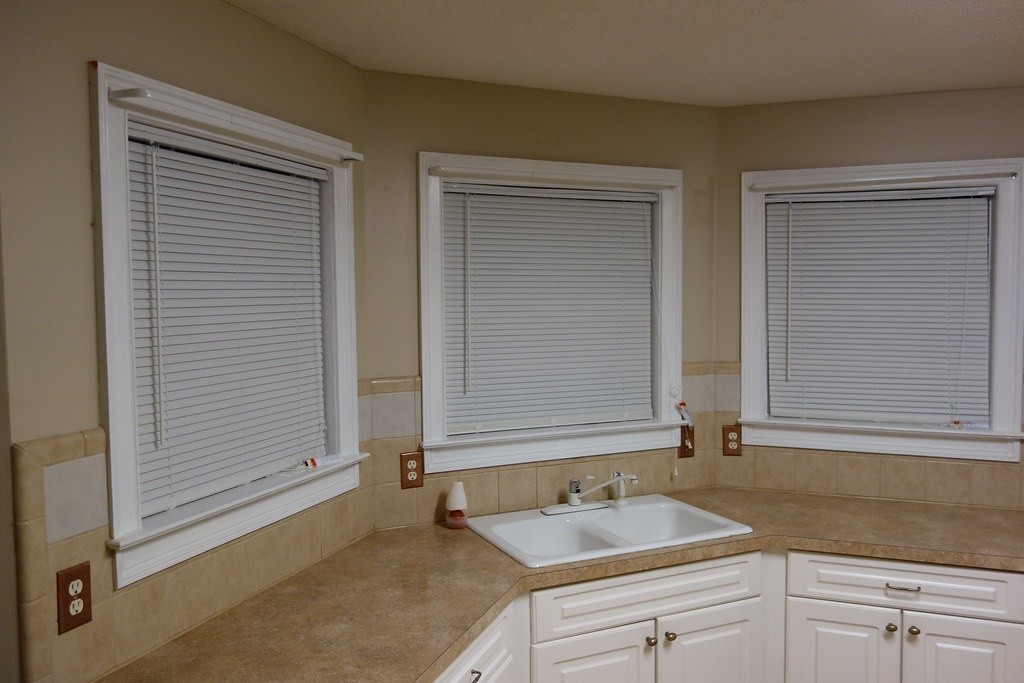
[466,506,633,569]
[588,494,754,548]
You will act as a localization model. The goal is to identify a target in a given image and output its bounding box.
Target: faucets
[568,473,639,505]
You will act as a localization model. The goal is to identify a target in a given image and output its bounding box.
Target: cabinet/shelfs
[530,550,762,683]
[429,601,521,683]
[786,548,1024,682]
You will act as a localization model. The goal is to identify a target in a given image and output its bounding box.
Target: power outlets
[722,425,742,456]
[56,561,92,636]
[400,451,423,489]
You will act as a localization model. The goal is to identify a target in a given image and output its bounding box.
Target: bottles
[445,481,468,530]
